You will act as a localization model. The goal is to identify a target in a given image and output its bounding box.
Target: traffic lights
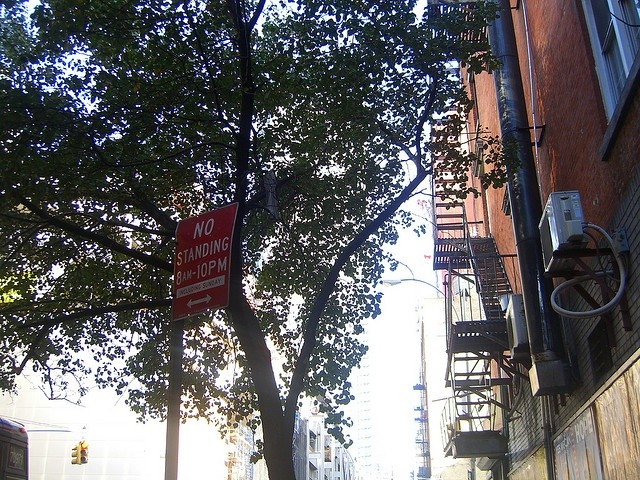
[80,442,88,463]
[71,444,80,464]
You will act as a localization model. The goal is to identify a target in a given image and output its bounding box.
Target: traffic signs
[171,202,239,321]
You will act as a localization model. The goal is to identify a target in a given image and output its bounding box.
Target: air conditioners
[538,190,588,272]
[505,294,529,359]
